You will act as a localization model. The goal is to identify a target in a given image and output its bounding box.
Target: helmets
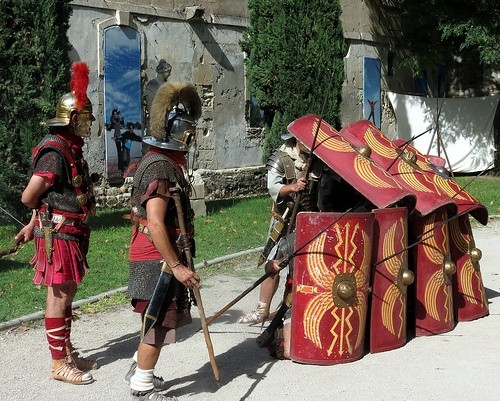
[281,133,293,140]
[46,91,96,126]
[142,108,198,151]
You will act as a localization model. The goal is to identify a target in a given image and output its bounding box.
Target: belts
[35,211,84,227]
[138,224,149,235]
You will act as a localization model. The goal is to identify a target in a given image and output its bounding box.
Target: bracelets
[192,281,198,285]
[170,261,180,268]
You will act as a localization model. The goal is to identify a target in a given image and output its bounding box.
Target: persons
[367,98,378,126]
[238,133,312,323]
[256,156,369,360]
[105,109,125,171]
[110,123,142,177]
[143,59,172,135]
[124,103,201,401]
[15,91,98,385]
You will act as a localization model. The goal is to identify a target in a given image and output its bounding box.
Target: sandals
[129,388,178,401]
[52,362,94,384]
[237,305,269,323]
[65,352,100,369]
[125,361,165,388]
